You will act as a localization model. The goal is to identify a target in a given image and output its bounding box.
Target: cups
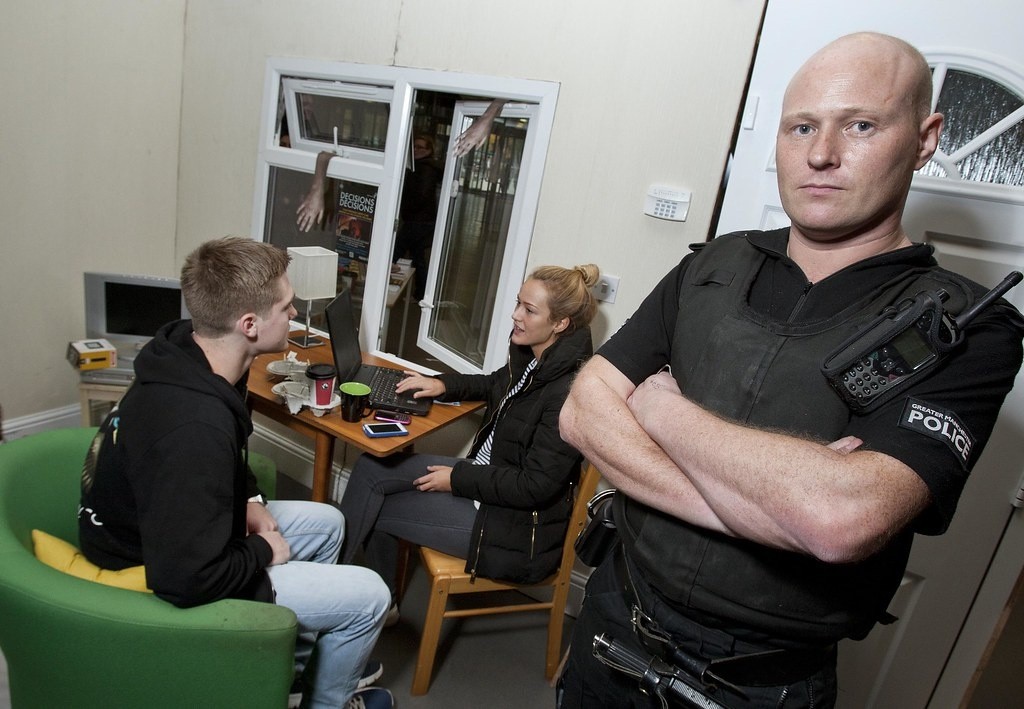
[305,363,338,406]
[339,382,374,423]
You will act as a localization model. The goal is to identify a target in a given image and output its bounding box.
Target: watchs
[247,494,268,507]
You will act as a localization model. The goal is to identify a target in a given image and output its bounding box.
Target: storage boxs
[65,339,118,372]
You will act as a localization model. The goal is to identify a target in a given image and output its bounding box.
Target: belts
[611,545,807,700]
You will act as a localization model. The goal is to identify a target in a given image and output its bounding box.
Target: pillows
[31,528,154,593]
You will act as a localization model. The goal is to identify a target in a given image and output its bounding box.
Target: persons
[295,150,338,232]
[451,99,510,159]
[554,31,1024,708]
[338,263,601,620]
[76,235,397,708]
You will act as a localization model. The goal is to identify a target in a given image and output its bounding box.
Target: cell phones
[374,409,411,425]
[363,423,409,438]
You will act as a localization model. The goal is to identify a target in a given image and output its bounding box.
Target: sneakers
[346,687,395,709]
[289,658,383,709]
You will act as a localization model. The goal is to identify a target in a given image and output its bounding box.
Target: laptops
[325,286,431,416]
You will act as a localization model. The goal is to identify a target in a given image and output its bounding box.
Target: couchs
[0,428,297,709]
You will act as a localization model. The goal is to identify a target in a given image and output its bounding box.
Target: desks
[76,381,130,427]
[238,327,488,503]
[348,266,417,359]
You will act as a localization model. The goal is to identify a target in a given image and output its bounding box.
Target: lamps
[286,246,339,349]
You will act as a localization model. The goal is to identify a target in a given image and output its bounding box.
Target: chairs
[392,459,602,696]
